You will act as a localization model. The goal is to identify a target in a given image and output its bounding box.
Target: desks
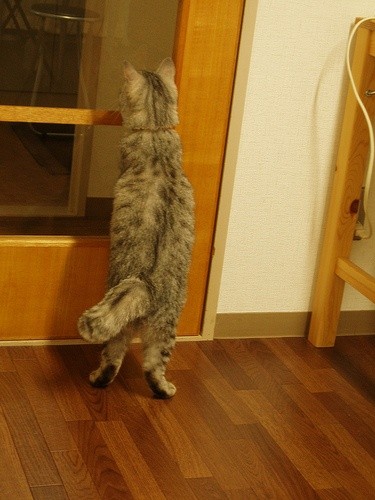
[306,16,375,349]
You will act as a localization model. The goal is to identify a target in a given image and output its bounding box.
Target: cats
[77,58,195,398]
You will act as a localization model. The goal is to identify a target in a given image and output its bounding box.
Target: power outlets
[353,187,365,240]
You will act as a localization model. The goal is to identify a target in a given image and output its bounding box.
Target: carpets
[11,124,73,176]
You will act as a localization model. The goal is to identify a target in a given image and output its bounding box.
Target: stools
[11,3,105,138]
